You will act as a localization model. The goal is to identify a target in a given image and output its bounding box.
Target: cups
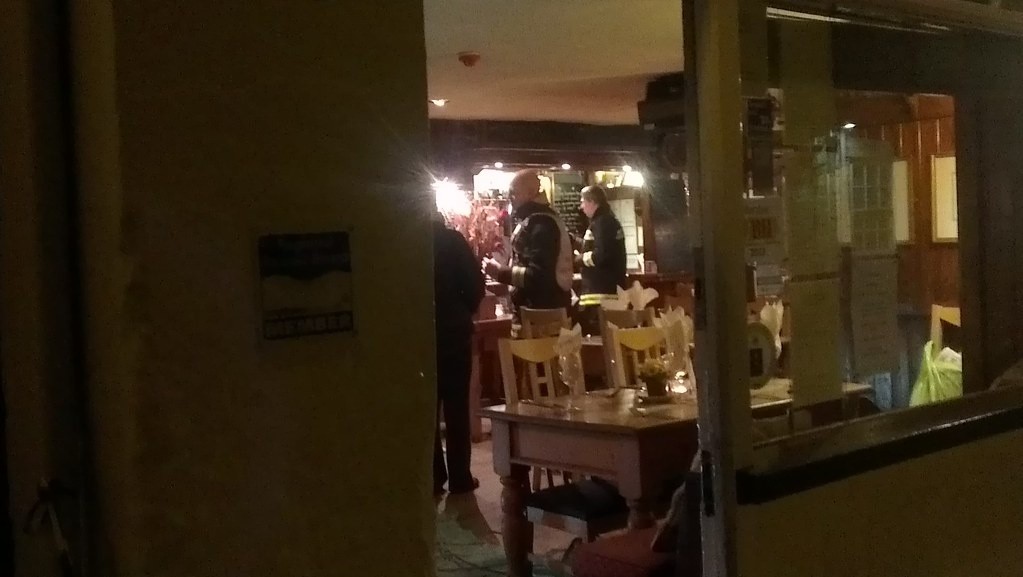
[495,303,504,320]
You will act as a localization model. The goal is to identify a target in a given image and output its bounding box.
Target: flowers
[638,360,670,381]
[440,201,499,254]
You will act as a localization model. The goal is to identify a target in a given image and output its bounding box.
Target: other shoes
[448,478,478,494]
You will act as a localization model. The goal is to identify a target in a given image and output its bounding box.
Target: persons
[482,171,574,400]
[568,186,627,390]
[432,199,486,493]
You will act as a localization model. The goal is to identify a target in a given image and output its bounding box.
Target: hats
[513,170,540,195]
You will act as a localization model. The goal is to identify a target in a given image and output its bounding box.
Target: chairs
[499,306,695,543]
[572,387,875,576]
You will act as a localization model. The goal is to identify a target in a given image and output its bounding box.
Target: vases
[646,376,666,396]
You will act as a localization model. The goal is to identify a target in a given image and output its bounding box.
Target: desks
[469,271,873,577]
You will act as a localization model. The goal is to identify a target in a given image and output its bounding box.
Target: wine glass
[557,353,584,412]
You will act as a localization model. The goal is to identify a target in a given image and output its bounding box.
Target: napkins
[760,299,784,359]
[554,322,582,385]
[617,280,659,310]
[652,305,684,357]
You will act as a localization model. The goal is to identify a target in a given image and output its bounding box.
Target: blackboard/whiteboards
[552,174,583,239]
